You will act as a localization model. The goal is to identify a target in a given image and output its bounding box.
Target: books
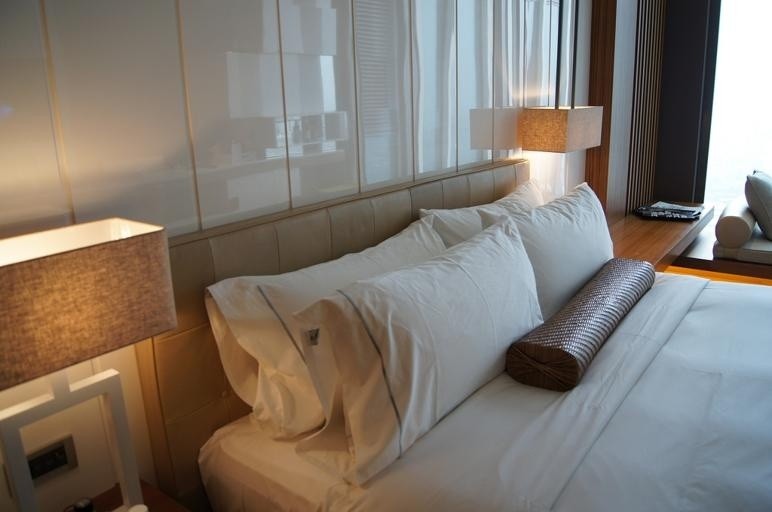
[633,201,705,221]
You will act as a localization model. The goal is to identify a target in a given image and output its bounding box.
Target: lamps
[1,214,180,511]
[518,1,604,154]
[469,0,523,151]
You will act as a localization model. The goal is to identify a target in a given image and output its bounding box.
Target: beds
[134,157,772,512]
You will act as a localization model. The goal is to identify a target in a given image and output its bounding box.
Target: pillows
[506,259,655,391]
[294,212,545,487]
[418,178,545,248]
[204,214,449,445]
[715,169,771,249]
[476,177,614,326]
